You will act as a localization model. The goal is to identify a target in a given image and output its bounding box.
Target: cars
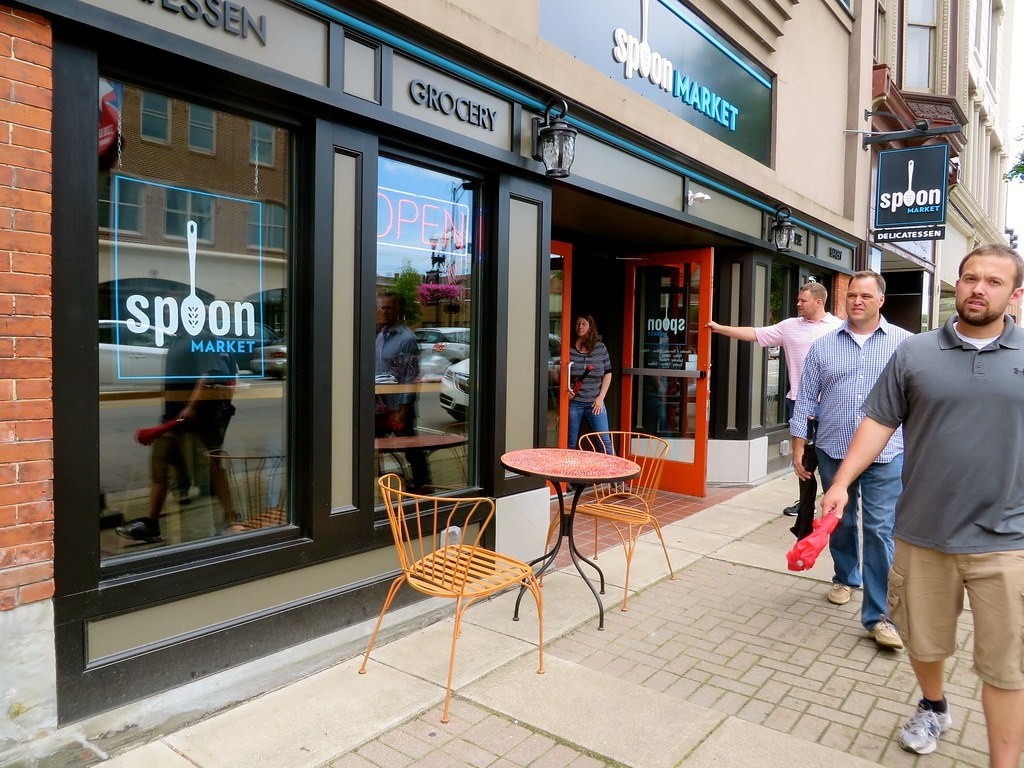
[249,330,286,380]
[412,326,472,384]
[439,359,473,426]
[99,319,175,401]
[200,320,281,370]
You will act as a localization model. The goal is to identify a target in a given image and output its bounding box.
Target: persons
[559,313,633,495]
[117,296,238,539]
[788,270,916,649]
[820,244,1023,768]
[705,282,845,516]
[375,292,437,497]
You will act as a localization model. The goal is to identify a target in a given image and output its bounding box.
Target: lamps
[688,190,711,208]
[913,117,935,130]
[531,99,578,178]
[768,206,797,252]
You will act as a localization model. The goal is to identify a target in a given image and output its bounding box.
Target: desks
[501,449,641,631]
[374,435,468,498]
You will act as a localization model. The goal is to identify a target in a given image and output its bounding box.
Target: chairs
[204,449,288,532]
[359,473,542,723]
[538,431,675,613]
[425,422,472,490]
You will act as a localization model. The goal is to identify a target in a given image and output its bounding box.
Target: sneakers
[898,700,951,754]
[212,512,243,537]
[116,522,162,542]
[610,482,633,493]
[783,500,817,516]
[567,483,573,491]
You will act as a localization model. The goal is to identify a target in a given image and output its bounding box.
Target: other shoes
[867,614,903,649]
[827,582,856,604]
[199,485,217,496]
[174,492,191,504]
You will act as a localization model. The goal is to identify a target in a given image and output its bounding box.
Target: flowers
[420,284,459,305]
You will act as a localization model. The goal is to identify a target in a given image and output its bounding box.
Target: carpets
[579,488,645,505]
